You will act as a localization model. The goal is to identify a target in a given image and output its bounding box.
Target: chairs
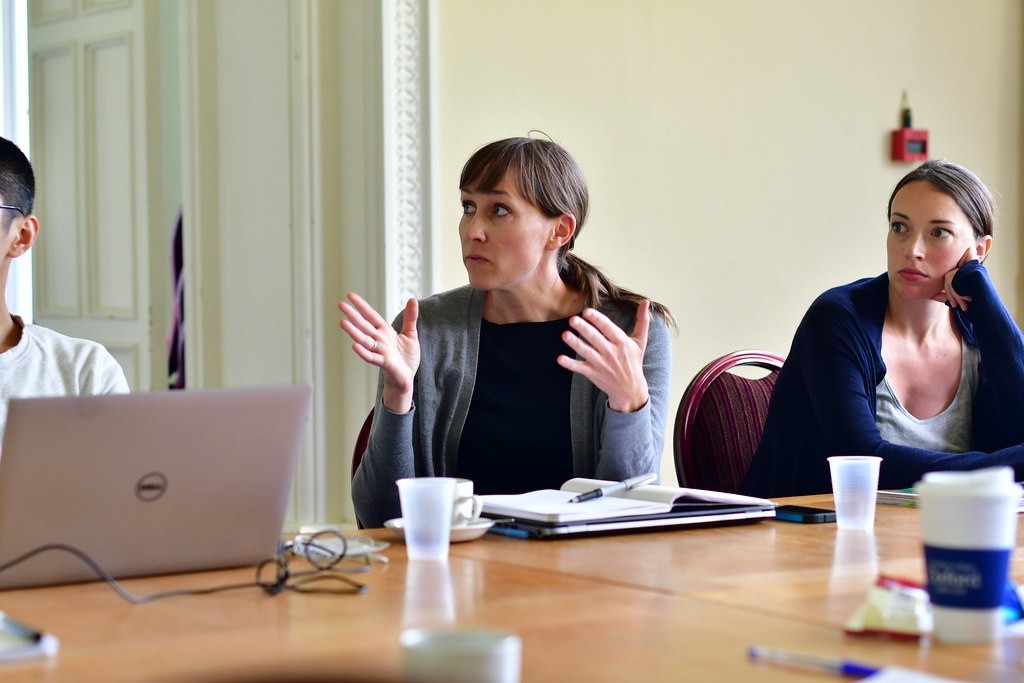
[673,350,788,494]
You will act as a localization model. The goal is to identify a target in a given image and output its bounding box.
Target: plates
[384,518,495,542]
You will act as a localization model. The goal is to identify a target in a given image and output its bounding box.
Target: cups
[827,456,883,530]
[914,465,1022,644]
[451,479,483,527]
[395,477,455,562]
[402,628,521,683]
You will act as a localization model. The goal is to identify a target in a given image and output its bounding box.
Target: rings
[371,341,378,351]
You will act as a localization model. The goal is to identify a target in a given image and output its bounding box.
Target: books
[876,482,1024,512]
[475,475,778,522]
[0,609,60,664]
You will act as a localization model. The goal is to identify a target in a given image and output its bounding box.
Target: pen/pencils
[0,610,41,643]
[896,487,919,493]
[749,644,876,677]
[567,472,658,503]
[490,526,529,539]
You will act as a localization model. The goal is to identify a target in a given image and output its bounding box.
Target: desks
[0,491,1024,683]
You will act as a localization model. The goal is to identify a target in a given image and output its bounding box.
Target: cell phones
[775,504,837,524]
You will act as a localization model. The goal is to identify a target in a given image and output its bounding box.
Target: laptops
[481,502,775,541]
[0,382,314,591]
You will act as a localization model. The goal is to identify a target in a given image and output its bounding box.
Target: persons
[741,154,1024,485]
[0,136,129,457]
[337,135,671,533]
